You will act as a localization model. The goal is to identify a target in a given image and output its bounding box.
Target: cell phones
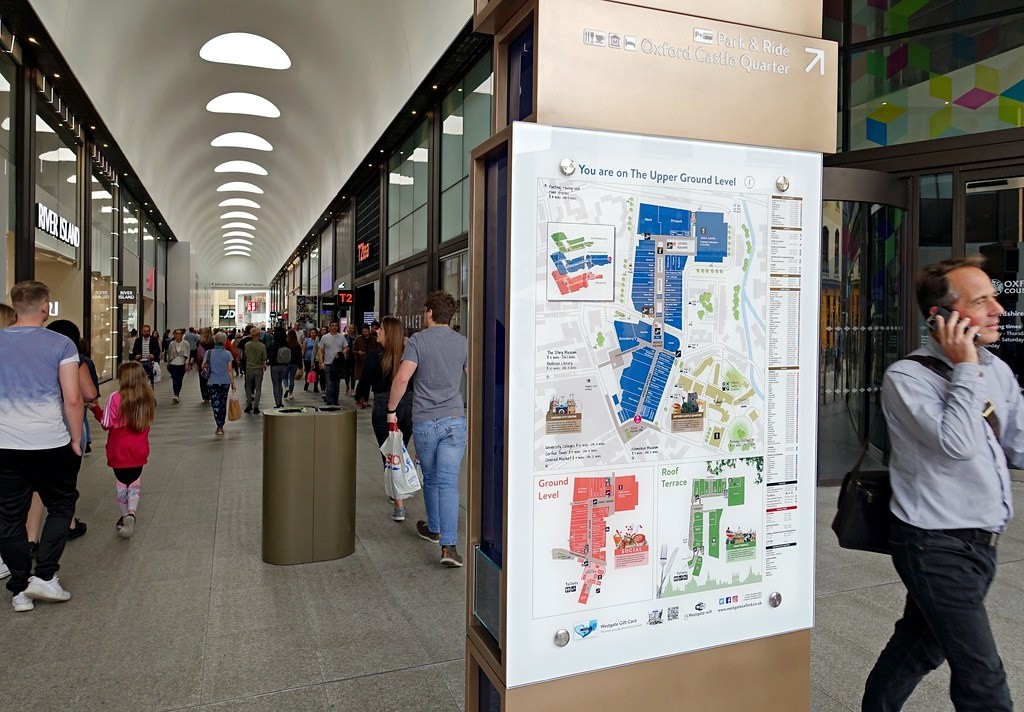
[929,307,981,342]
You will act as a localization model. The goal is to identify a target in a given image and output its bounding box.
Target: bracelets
[388,408,397,414]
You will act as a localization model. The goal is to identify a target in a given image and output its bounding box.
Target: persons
[0,303,17,581]
[92,328,108,378]
[354,317,414,521]
[25,320,98,559]
[79,338,102,456]
[86,362,155,540]
[127,320,379,437]
[386,292,468,569]
[861,256,1024,712]
[0,281,85,613]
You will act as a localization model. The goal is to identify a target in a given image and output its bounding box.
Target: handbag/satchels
[228,390,242,421]
[201,349,212,379]
[379,421,421,500]
[830,469,899,555]
[307,366,316,383]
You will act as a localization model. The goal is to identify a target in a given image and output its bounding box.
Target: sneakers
[12,591,34,611]
[439,544,463,567]
[67,518,86,541]
[24,575,71,601]
[0,556,12,578]
[28,539,39,560]
[417,520,440,543]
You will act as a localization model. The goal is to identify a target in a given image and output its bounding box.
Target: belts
[938,527,999,548]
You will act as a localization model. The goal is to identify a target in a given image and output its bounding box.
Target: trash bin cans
[263,403,357,565]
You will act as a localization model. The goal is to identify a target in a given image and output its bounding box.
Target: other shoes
[253,408,260,414]
[215,427,223,435]
[116,515,124,530]
[274,384,372,409]
[244,406,253,412]
[391,507,405,521]
[172,394,180,404]
[389,496,395,504]
[119,513,136,538]
[85,445,91,456]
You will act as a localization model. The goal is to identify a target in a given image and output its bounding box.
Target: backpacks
[277,346,291,364]
